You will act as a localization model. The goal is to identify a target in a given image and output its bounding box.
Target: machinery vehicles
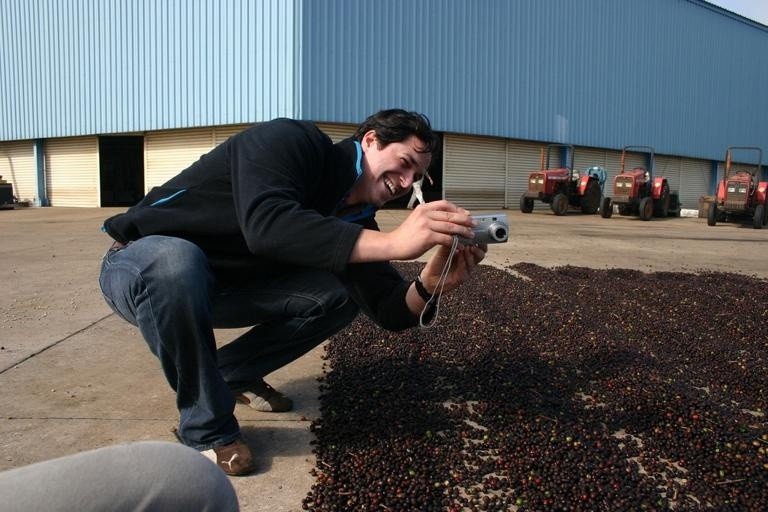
[523,141,607,214]
[598,145,681,220]
[698,144,767,230]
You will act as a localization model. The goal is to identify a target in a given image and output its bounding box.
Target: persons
[99,110,488,475]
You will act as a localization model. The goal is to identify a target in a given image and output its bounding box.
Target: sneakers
[200,438,255,476]
[234,382,293,413]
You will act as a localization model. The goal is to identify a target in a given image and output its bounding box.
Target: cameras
[454,215,508,248]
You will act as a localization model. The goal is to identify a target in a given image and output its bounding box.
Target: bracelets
[414,274,434,307]
